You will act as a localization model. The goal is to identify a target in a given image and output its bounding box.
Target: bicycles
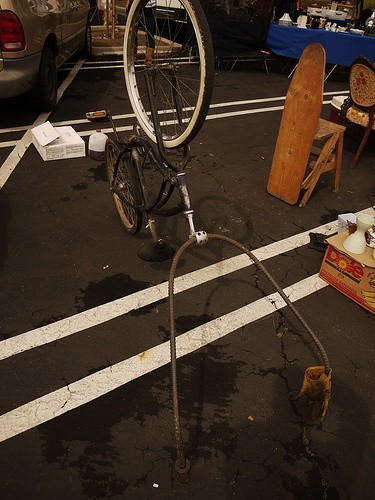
[84,0,218,266]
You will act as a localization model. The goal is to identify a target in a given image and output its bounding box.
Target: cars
[0,1,97,114]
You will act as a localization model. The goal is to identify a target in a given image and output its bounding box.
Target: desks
[264,22,375,84]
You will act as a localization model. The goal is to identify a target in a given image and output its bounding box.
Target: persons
[124,0,156,68]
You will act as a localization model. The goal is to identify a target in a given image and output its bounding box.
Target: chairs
[333,54,375,170]
[266,42,347,208]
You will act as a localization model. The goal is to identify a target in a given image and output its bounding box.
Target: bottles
[88,129,108,161]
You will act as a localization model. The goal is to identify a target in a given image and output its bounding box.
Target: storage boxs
[326,10,347,21]
[318,230,375,314]
[307,7,327,17]
[30,120,86,162]
[328,95,351,127]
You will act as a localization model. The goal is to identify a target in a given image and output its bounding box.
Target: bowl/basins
[350,29,365,35]
